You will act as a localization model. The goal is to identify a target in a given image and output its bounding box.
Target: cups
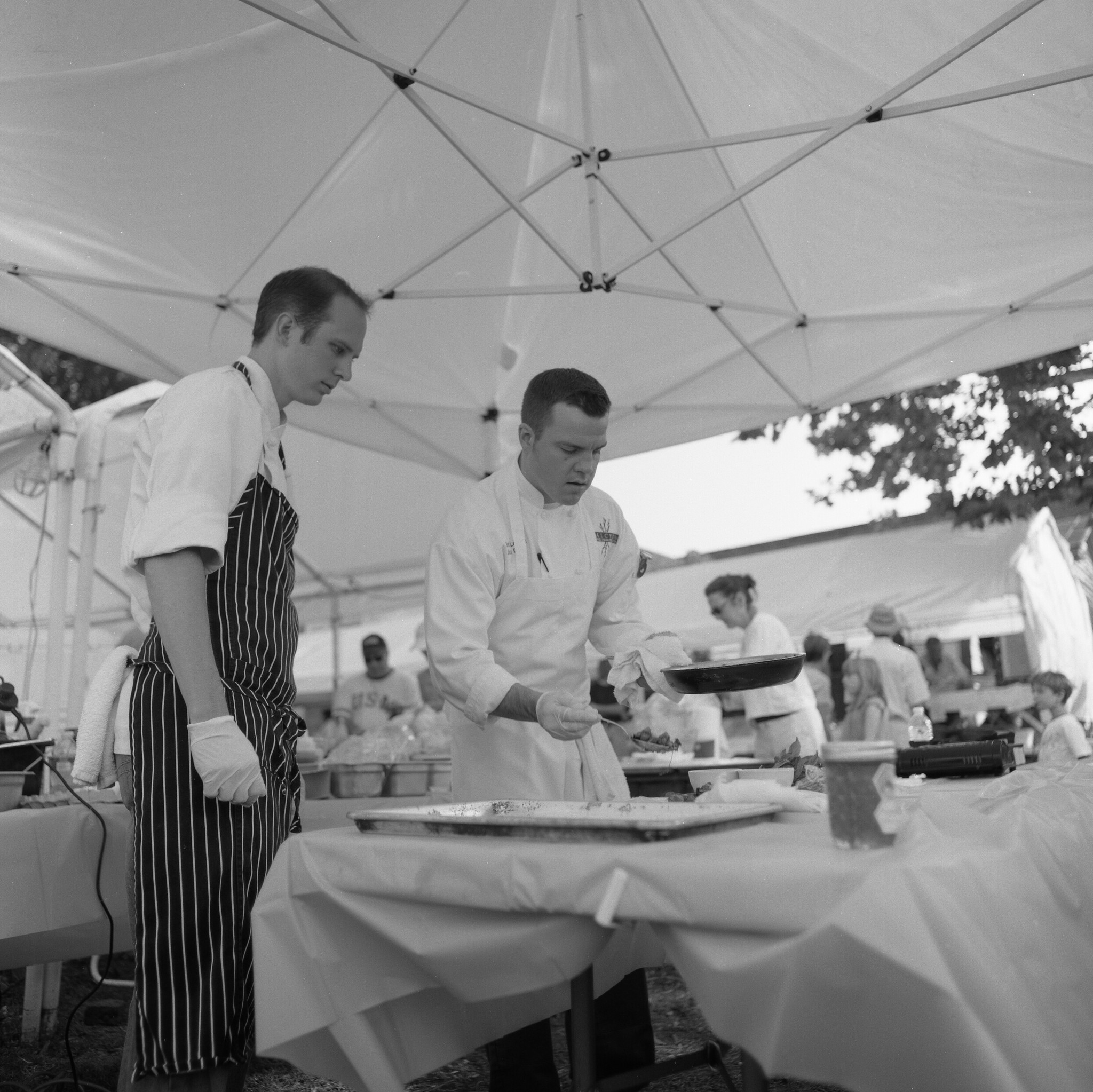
[821,740,898,849]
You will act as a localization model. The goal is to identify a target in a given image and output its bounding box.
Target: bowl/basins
[688,768,741,794]
[0,771,34,812]
[660,652,807,695]
[738,768,795,788]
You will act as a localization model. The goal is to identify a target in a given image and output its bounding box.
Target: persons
[333,635,425,733]
[1032,670,1093,760]
[423,368,970,1092]
[112,263,372,1092]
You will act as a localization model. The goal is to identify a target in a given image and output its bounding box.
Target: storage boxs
[297,754,453,803]
[814,736,904,856]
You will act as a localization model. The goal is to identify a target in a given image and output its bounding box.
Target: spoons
[602,718,677,752]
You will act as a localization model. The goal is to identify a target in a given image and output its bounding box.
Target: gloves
[188,716,266,805]
[534,690,602,741]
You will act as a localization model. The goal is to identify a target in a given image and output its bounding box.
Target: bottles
[907,706,934,748]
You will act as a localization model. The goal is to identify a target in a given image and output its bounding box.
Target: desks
[0,799,145,1070]
[618,752,780,800]
[263,781,1092,1092]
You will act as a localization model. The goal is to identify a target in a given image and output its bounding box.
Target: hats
[866,607,900,634]
[363,635,384,650]
[805,633,830,662]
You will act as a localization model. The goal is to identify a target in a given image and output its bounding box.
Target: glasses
[711,593,735,616]
[365,655,382,663]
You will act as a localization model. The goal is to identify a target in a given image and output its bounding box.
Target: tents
[0,345,486,1045]
